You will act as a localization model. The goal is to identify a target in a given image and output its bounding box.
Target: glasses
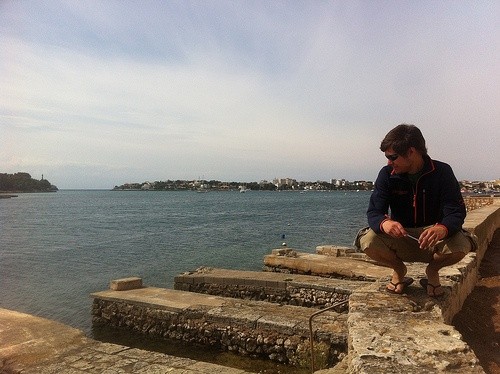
[386,153,400,161]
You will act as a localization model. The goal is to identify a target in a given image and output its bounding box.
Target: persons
[354,124,467,296]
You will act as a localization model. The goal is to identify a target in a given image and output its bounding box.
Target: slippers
[420,278,445,297]
[386,276,413,294]
